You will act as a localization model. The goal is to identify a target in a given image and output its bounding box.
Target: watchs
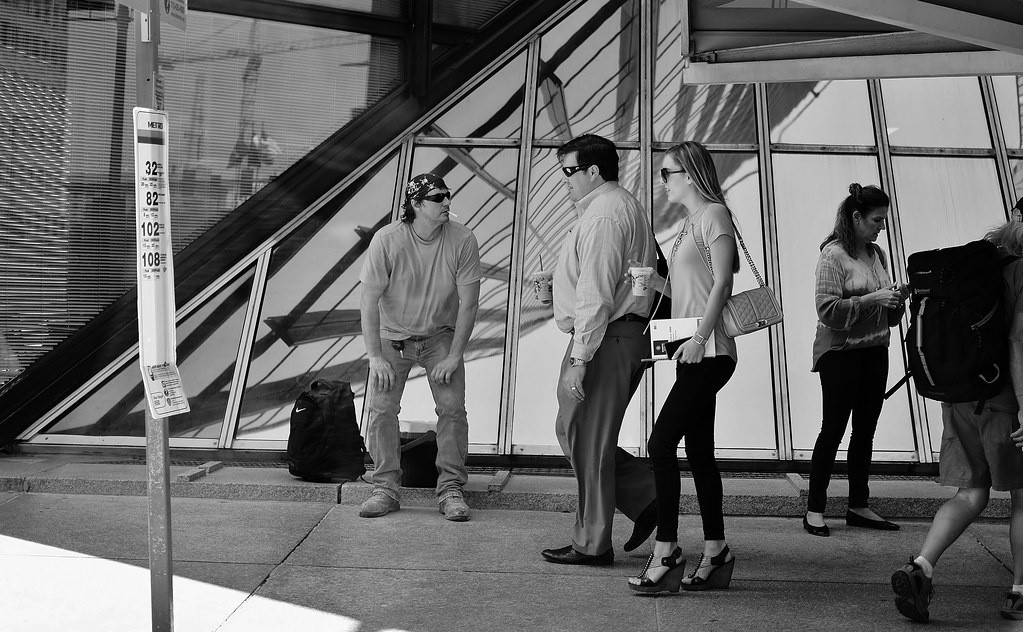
[569,357,587,367]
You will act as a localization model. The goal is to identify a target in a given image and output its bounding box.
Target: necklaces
[688,199,707,220]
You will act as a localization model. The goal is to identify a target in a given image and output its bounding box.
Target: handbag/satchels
[649,259,672,319]
[722,286,782,338]
[400,430,438,488]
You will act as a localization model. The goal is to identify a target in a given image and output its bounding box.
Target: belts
[567,313,649,335]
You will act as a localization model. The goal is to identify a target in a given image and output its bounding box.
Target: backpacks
[885,239,1023,416]
[287,379,372,504]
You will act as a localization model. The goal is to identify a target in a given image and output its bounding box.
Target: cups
[531,271,553,301]
[629,267,653,297]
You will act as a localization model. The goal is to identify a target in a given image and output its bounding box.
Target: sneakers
[360,491,400,517]
[1000,590,1023,620]
[439,496,471,520]
[891,555,935,622]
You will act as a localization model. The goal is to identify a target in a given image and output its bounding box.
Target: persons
[804,183,912,536]
[359,173,483,521]
[890,199,1023,623]
[542,135,658,567]
[624,141,743,592]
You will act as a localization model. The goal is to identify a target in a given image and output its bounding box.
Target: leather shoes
[624,498,659,552]
[541,545,615,567]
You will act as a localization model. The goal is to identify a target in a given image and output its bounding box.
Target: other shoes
[846,509,901,531]
[803,511,830,537]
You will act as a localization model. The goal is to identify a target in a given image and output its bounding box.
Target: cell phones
[665,337,693,360]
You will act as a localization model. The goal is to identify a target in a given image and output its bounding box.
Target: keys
[392,340,405,359]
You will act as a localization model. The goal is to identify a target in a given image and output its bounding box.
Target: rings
[571,386,576,390]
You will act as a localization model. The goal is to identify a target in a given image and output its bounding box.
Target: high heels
[628,545,686,593]
[680,544,735,591]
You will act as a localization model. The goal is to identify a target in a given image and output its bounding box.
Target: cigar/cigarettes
[447,210,458,218]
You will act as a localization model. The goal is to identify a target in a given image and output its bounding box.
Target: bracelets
[692,331,710,346]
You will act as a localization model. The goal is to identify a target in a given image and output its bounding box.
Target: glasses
[562,164,592,177]
[422,192,450,203]
